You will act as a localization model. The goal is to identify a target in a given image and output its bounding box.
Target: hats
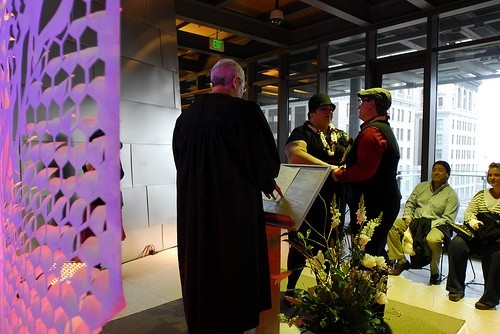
[309,94,336,113]
[357,88,391,110]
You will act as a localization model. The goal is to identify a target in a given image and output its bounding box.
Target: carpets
[98,266,467,334]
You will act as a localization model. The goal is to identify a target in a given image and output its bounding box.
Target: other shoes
[449,292,464,301]
[475,301,493,310]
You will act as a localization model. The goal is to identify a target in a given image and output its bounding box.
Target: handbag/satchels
[463,212,500,244]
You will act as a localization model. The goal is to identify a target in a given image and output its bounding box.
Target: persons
[387,161,459,285]
[331,88,402,327]
[446,163,500,310]
[171,58,284,334]
[81,129,126,295]
[283,93,354,308]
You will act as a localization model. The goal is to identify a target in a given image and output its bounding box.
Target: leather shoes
[388,260,411,276]
[430,273,441,285]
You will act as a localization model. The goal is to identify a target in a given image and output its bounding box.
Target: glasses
[358,98,369,106]
[238,77,248,92]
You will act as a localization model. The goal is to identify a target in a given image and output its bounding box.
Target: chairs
[399,238,451,283]
[465,255,485,286]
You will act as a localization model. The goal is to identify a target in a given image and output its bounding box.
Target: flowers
[306,116,340,156]
[277,194,398,334]
